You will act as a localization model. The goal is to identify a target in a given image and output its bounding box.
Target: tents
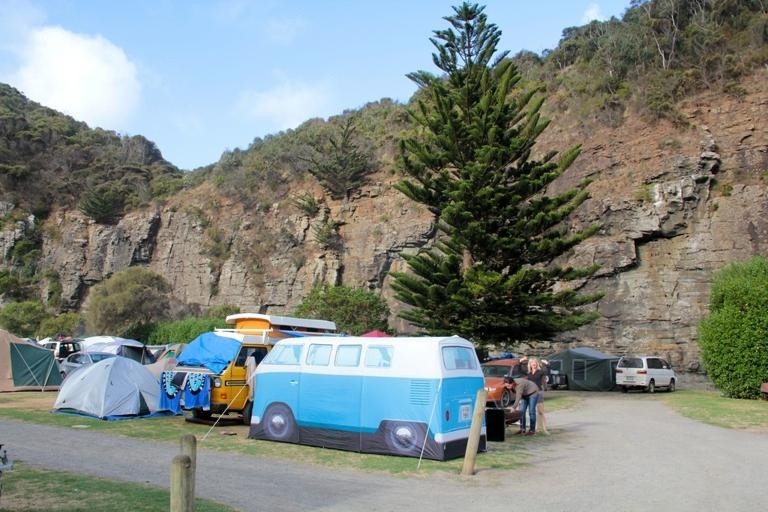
[246,335,487,461]
[546,348,618,391]
[0,327,64,393]
[53,356,162,419]
[18,333,187,364]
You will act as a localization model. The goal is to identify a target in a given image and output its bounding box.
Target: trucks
[163,314,337,425]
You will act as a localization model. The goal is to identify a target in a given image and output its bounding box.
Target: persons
[501,377,539,436]
[524,356,552,438]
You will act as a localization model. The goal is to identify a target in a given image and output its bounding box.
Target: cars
[478,358,548,408]
[55,353,123,382]
[40,337,84,364]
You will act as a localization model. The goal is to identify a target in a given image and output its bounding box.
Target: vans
[614,355,679,393]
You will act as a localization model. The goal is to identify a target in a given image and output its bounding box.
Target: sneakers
[515,430,536,437]
